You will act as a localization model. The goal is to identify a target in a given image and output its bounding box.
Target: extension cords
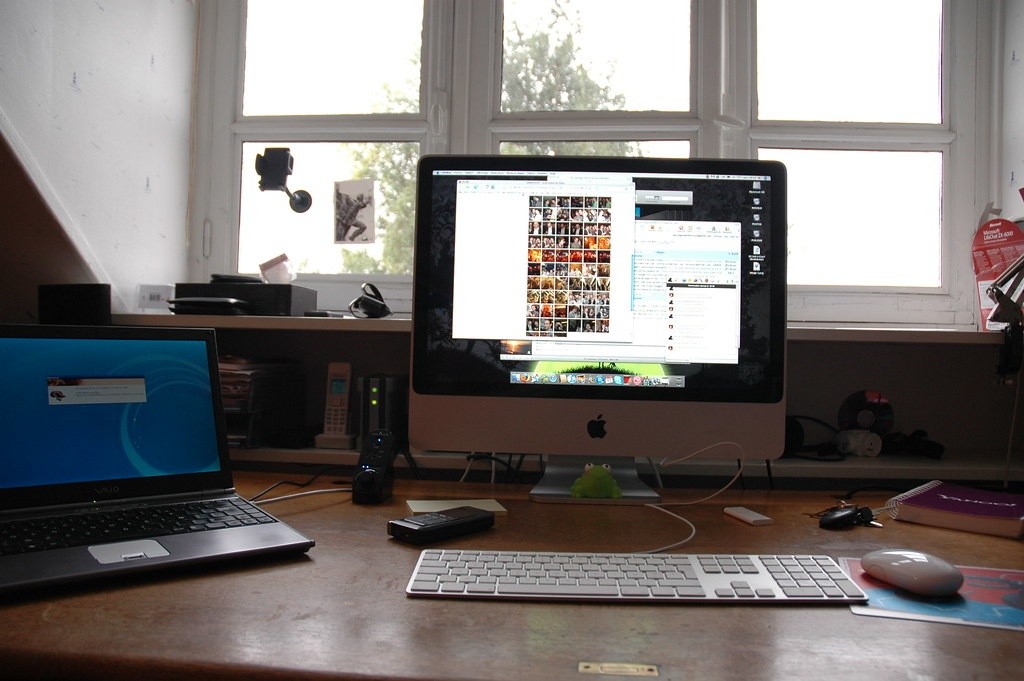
[837,428,882,457]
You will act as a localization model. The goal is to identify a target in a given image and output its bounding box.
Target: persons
[526,196,611,337]
[334,182,371,242]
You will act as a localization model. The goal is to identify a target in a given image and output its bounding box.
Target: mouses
[861,548,964,597]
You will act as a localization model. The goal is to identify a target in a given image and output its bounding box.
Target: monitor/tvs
[409,155,787,505]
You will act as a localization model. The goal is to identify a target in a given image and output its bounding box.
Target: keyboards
[406,550,867,605]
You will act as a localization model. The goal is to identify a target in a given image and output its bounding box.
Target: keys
[802,500,896,528]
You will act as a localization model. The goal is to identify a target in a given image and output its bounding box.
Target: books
[217,354,307,448]
[885,480,1024,539]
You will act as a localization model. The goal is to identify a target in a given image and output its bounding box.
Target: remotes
[724,506,773,526]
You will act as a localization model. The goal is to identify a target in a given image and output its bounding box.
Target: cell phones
[387,505,495,543]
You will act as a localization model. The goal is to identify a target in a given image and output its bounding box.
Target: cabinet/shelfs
[108,312,1024,484]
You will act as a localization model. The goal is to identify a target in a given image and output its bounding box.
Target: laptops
[0,324,316,600]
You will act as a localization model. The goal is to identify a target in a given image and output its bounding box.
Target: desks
[0,470,1024,681]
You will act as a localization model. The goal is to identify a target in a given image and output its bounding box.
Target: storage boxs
[171,281,319,316]
